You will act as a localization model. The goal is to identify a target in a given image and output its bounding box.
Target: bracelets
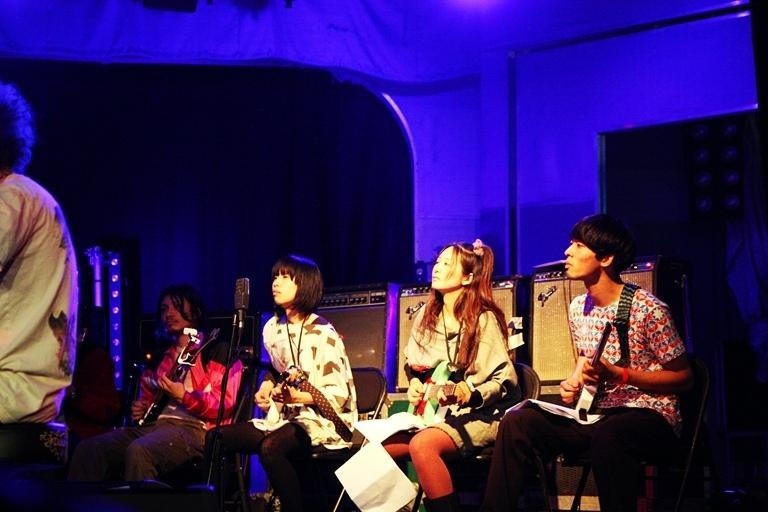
[616,367,629,386]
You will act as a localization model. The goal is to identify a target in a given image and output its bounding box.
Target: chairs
[563,353,711,512]
[413,363,551,512]
[234,366,387,512]
[108,338,259,511]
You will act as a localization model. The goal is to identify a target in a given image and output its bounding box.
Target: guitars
[265,367,296,424]
[408,360,471,424]
[138,328,220,426]
[576,322,612,413]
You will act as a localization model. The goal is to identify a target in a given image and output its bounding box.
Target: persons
[66,283,245,483]
[381,238,522,512]
[484,214,696,512]
[1,81,80,512]
[201,256,359,512]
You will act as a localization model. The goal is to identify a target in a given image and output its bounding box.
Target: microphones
[233,277,250,329]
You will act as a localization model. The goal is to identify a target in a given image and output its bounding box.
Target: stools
[0,421,70,512]
